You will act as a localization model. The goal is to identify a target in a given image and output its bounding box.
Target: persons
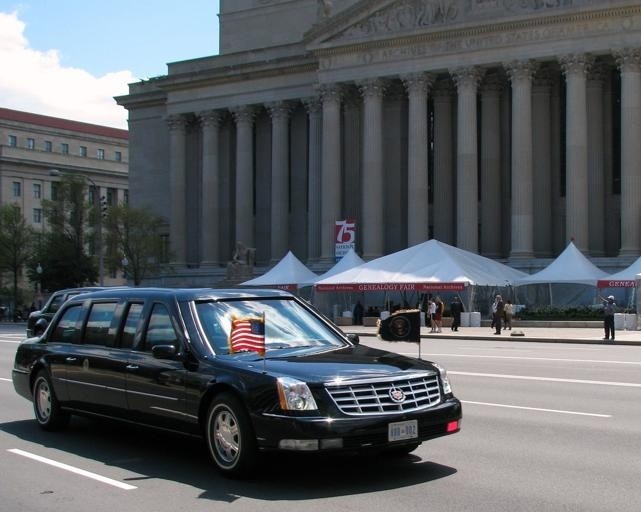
[598,294,616,339]
[503,300,514,330]
[353,299,364,324]
[433,295,445,333]
[450,296,463,331]
[0,299,44,322]
[490,298,498,328]
[426,297,437,333]
[493,294,505,334]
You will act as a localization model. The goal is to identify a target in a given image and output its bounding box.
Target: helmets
[608,295,615,301]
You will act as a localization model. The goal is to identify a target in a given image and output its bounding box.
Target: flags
[229,315,265,357]
